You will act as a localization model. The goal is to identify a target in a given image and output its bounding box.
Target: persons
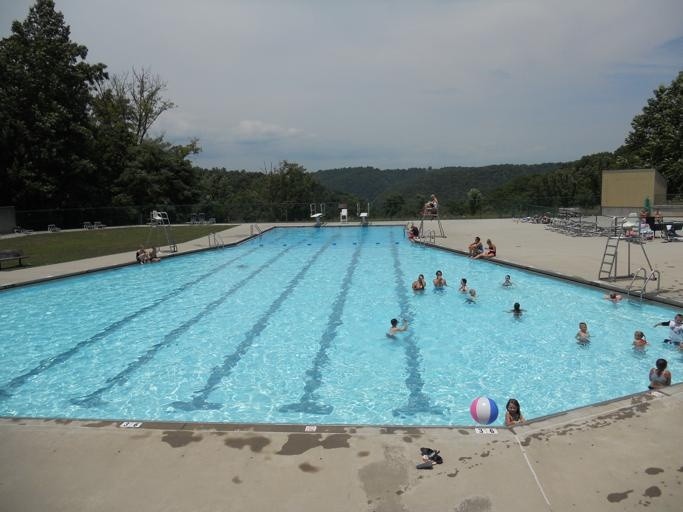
[433,270,476,307]
[605,293,622,308]
[633,313,683,390]
[473,239,496,259]
[149,247,160,262]
[575,322,591,350]
[419,194,438,213]
[654,209,662,224]
[502,275,511,286]
[505,399,524,426]
[136,244,148,264]
[407,224,418,239]
[412,274,426,291]
[640,209,648,223]
[468,236,483,258]
[386,318,407,340]
[503,303,527,322]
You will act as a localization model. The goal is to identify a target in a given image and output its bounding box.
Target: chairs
[422,201,439,217]
[512,212,681,243]
[9,219,106,236]
[145,208,217,227]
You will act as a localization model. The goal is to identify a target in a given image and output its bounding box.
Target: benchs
[0,247,31,272]
